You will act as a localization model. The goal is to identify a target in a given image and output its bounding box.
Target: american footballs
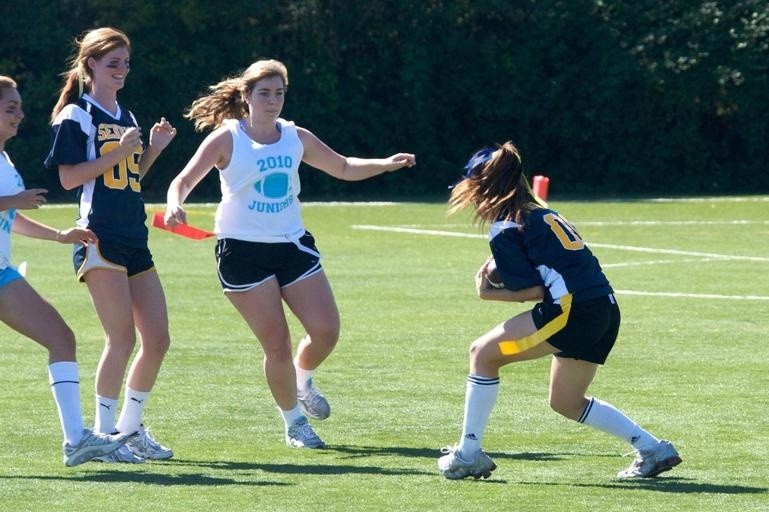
[483,257,504,291]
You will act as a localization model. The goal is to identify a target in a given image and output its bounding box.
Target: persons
[162,57,421,451]
[38,23,180,467]
[433,137,683,482]
[0,69,131,468]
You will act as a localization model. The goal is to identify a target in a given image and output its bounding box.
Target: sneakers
[115,424,174,461]
[616,439,683,480]
[89,429,146,464]
[296,374,330,420]
[62,427,128,467]
[437,448,497,481]
[286,416,327,450]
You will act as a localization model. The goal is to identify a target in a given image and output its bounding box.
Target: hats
[447,147,500,188]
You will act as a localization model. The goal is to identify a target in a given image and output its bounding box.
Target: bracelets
[56,228,62,242]
